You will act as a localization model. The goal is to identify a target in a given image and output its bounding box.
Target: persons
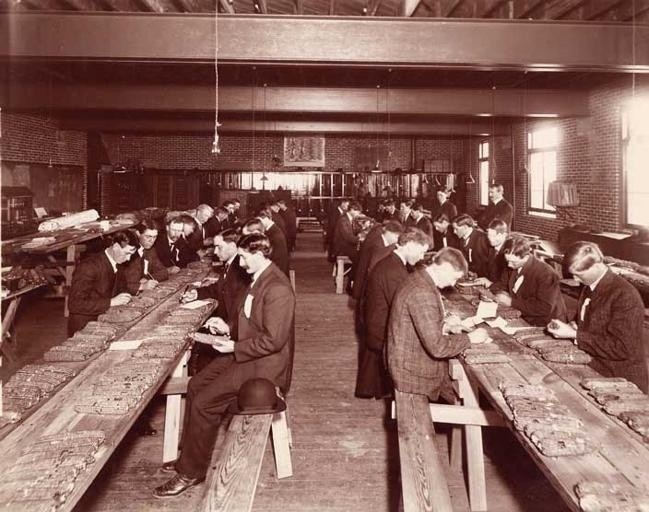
[151,232,300,501]
[366,227,434,351]
[377,199,512,285]
[387,249,490,403]
[126,198,241,295]
[178,227,249,373]
[328,201,402,316]
[546,240,649,395]
[489,237,567,327]
[431,188,458,223]
[235,198,297,277]
[477,183,515,233]
[67,230,141,337]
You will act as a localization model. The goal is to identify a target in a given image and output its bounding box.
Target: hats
[227,378,286,416]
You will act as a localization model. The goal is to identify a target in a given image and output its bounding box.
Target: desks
[2,220,648,512]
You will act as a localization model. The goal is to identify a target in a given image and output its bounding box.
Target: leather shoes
[152,473,205,498]
[133,422,158,436]
[160,461,178,473]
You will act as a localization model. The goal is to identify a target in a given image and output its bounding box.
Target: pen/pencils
[201,321,218,328]
[179,284,190,304]
[472,319,479,329]
[147,271,160,287]
[169,258,177,266]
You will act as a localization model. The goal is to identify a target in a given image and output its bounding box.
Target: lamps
[248,67,268,193]
[372,69,475,192]
